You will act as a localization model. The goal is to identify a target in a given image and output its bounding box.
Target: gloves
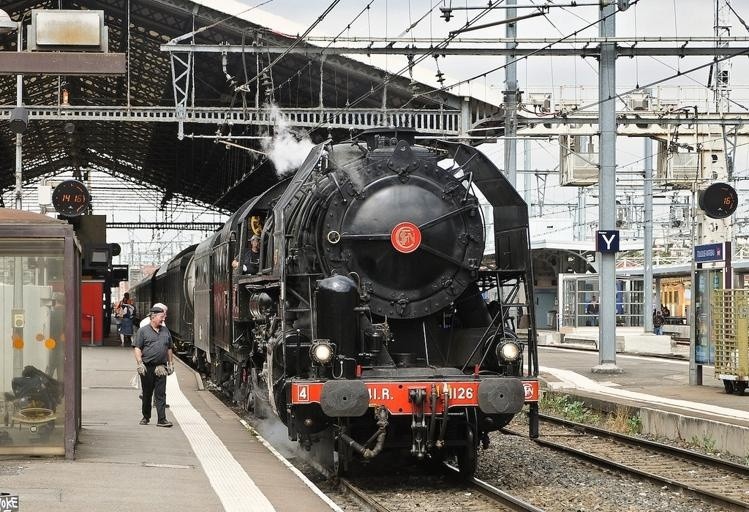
[155,365,167,377]
[136,360,148,376]
[168,362,174,375]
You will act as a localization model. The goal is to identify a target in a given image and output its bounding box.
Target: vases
[12,407,57,443]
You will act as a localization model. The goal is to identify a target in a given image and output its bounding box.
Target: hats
[146,307,164,316]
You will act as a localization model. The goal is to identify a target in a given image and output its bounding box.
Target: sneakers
[158,421,173,427]
[140,417,149,425]
[120,344,124,347]
[139,394,144,399]
[153,402,169,407]
[131,343,136,347]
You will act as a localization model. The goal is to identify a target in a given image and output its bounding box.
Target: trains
[125,126,538,484]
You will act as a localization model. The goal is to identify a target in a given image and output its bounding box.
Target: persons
[44,294,66,404]
[653,304,671,334]
[586,295,599,326]
[137,302,175,408]
[110,292,137,347]
[130,307,176,427]
[232,234,261,275]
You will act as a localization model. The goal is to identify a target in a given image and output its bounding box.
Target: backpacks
[123,304,136,319]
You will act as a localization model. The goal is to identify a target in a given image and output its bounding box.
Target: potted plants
[718,367,748,395]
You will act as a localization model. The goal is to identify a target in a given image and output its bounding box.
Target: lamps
[9,107,28,135]
